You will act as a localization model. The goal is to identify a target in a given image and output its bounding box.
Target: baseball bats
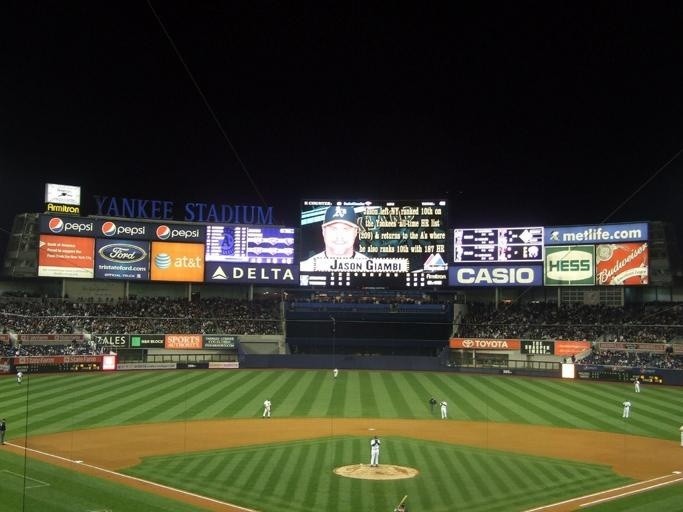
[393,495,407,512]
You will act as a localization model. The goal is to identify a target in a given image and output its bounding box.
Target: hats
[321,205,359,229]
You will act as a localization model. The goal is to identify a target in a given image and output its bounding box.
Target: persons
[299,207,371,286]
[395,504,406,512]
[454,299,682,344]
[1,289,282,386]
[0,419,7,442]
[370,436,381,467]
[285,289,466,308]
[262,398,272,418]
[622,400,631,418]
[429,397,436,414]
[679,424,683,446]
[561,344,682,393]
[440,399,447,419]
